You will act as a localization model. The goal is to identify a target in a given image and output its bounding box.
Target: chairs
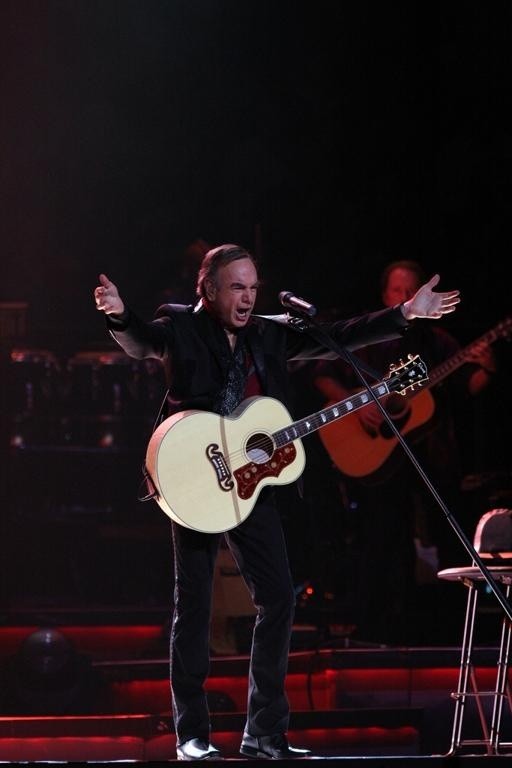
[432,507,512,757]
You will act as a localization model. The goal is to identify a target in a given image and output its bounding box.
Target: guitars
[146,353,430,534]
[319,319,512,477]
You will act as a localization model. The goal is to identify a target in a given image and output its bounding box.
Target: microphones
[279,291,317,316]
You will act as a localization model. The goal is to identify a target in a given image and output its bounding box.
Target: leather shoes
[177,737,221,761]
[240,730,312,759]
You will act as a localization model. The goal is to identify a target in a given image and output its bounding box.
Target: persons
[310,259,497,667]
[94,243,462,761]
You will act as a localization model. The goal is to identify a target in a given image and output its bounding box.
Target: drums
[11,351,59,425]
[77,351,138,425]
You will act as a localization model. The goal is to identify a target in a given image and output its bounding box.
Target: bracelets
[480,367,497,379]
